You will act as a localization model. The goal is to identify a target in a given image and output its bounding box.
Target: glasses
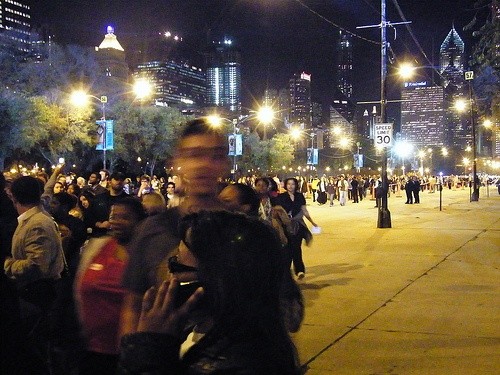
[168,256,199,274]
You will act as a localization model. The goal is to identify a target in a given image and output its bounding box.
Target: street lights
[71,92,107,170]
[233,109,272,183]
[398,64,479,201]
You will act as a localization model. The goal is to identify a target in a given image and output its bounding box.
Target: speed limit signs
[375,123,393,147]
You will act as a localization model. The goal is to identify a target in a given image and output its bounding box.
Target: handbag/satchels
[27,267,76,336]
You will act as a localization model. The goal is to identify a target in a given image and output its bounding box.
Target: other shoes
[298,272,305,281]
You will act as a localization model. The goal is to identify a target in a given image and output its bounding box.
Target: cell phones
[174,280,206,309]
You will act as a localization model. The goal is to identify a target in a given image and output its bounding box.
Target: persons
[0,120,317,375]
[309,170,500,208]
